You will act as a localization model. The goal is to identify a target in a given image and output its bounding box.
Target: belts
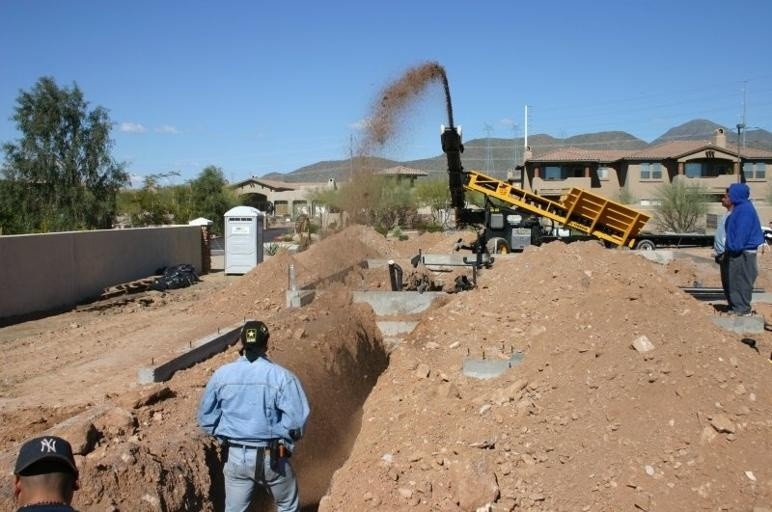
[231,444,270,450]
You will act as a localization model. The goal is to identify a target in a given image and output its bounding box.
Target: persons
[721,182,765,316]
[12,434,83,512]
[713,188,736,308]
[759,221,771,244]
[196,319,311,511]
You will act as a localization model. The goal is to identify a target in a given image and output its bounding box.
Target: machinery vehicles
[440,125,656,255]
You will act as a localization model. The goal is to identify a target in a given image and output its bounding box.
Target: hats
[14,436,78,476]
[242,320,269,361]
